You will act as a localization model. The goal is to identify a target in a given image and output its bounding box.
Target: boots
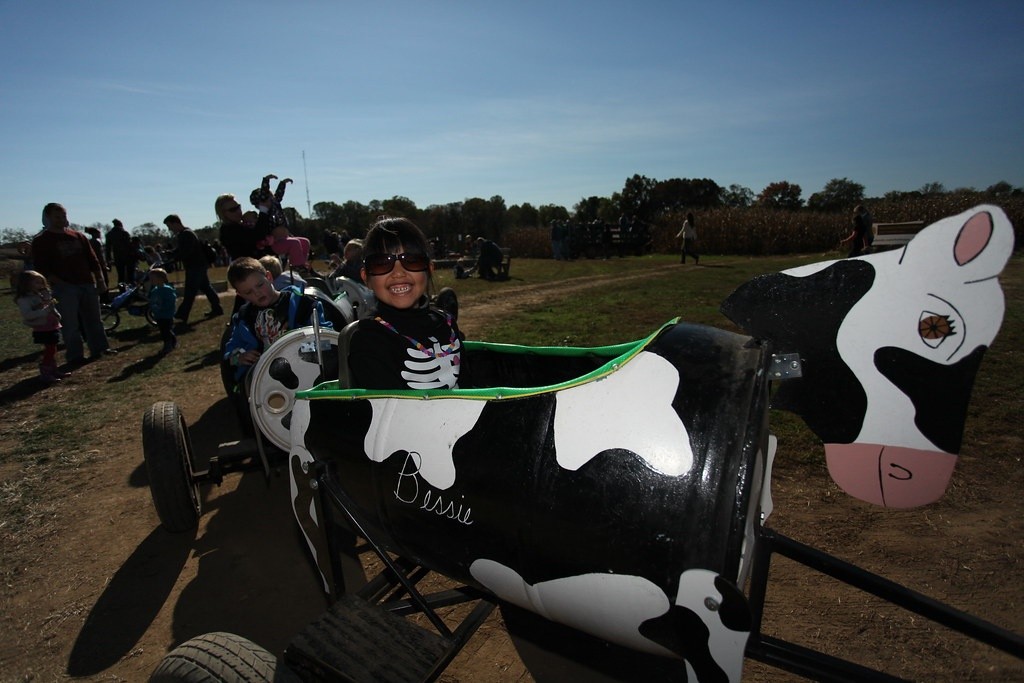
[49,363,72,377]
[40,367,62,382]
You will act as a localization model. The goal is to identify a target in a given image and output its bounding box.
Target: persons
[164,215,225,323]
[222,257,334,383]
[425,234,504,282]
[249,174,311,272]
[104,218,137,286]
[840,214,865,258]
[675,213,700,265]
[13,270,73,383]
[23,201,119,366]
[143,238,230,275]
[323,226,368,285]
[853,204,874,254]
[294,246,319,279]
[84,226,111,305]
[549,212,650,259]
[259,255,306,294]
[213,195,273,326]
[147,269,178,351]
[348,218,473,391]
[131,237,148,269]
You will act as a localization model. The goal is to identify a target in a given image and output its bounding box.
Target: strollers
[100,261,165,332]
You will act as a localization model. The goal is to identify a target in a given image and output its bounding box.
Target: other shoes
[204,308,223,316]
[173,313,188,321]
[68,357,93,365]
[292,265,308,274]
[91,349,118,356]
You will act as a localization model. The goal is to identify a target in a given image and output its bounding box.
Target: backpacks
[184,229,217,264]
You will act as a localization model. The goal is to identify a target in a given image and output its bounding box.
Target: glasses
[362,253,430,275]
[224,204,241,213]
